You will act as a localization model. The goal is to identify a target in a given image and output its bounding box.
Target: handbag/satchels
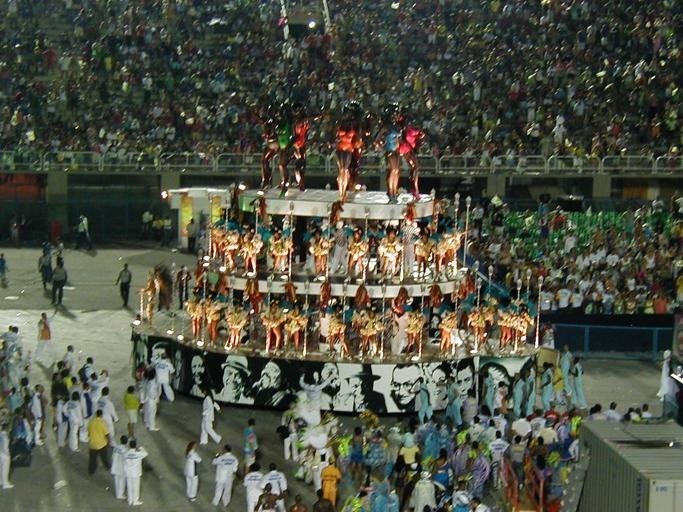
[275,416,292,439]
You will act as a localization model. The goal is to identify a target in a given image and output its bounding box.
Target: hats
[218,354,250,376]
[329,456,335,464]
[342,363,381,380]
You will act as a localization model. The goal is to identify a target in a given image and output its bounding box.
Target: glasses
[390,379,419,392]
